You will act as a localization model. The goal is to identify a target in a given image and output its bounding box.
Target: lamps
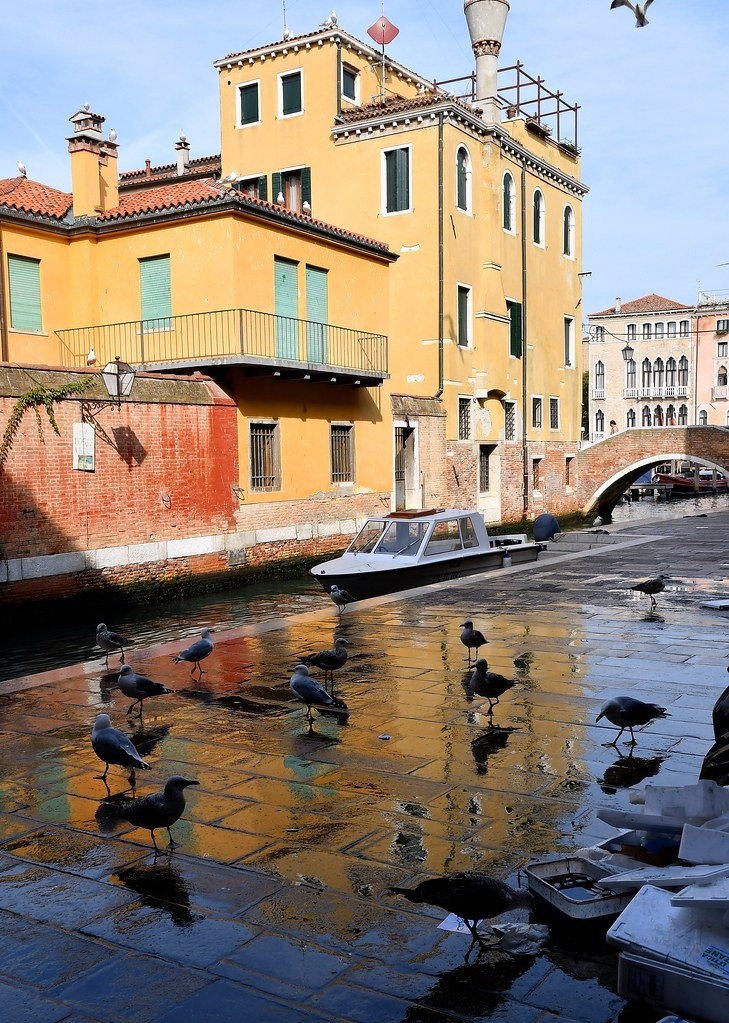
[582,324,634,363]
[80,356,136,422]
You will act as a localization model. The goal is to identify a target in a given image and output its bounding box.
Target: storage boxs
[523,779,729,1023]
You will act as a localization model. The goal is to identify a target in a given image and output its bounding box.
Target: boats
[310,508,544,598]
[652,465,729,497]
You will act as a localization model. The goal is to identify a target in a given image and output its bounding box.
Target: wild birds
[17,160,27,176]
[595,695,673,748]
[459,619,520,716]
[592,515,603,530]
[108,128,118,142]
[284,26,290,40]
[287,637,355,722]
[330,584,358,615]
[626,573,675,606]
[446,88,456,97]
[318,9,337,29]
[86,347,96,366]
[415,85,430,96]
[276,191,285,206]
[91,619,218,857]
[302,201,311,216]
[609,0,655,29]
[84,102,91,111]
[179,128,187,142]
[386,869,538,966]
[215,170,239,184]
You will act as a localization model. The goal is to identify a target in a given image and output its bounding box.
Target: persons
[610,420,618,435]
